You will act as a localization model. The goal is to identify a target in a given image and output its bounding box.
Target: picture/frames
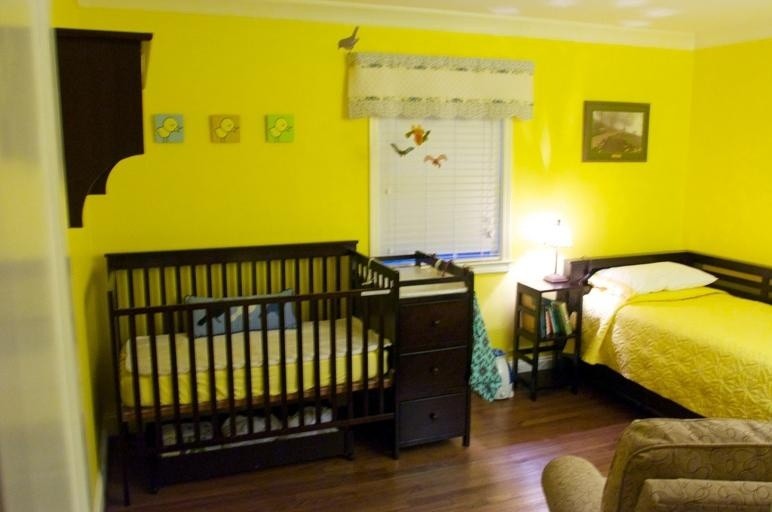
[582,101,651,161]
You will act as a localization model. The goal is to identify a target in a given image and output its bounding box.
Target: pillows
[186,288,297,337]
[588,262,718,300]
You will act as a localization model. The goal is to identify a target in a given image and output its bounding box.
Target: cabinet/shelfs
[53,27,153,226]
[357,250,473,459]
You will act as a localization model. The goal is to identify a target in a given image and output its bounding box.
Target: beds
[564,252,772,419]
[104,242,396,506]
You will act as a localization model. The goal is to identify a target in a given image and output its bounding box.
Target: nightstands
[513,281,583,401]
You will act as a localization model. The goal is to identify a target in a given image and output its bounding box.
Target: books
[540,298,572,337]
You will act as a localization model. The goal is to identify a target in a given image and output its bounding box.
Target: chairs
[540,418,772,511]
